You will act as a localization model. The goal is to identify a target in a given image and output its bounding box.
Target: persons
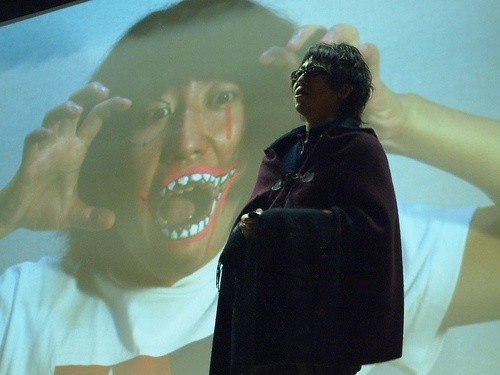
[0,0,500,375]
[209,45,404,375]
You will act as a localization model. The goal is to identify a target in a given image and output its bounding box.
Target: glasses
[291,65,330,81]
